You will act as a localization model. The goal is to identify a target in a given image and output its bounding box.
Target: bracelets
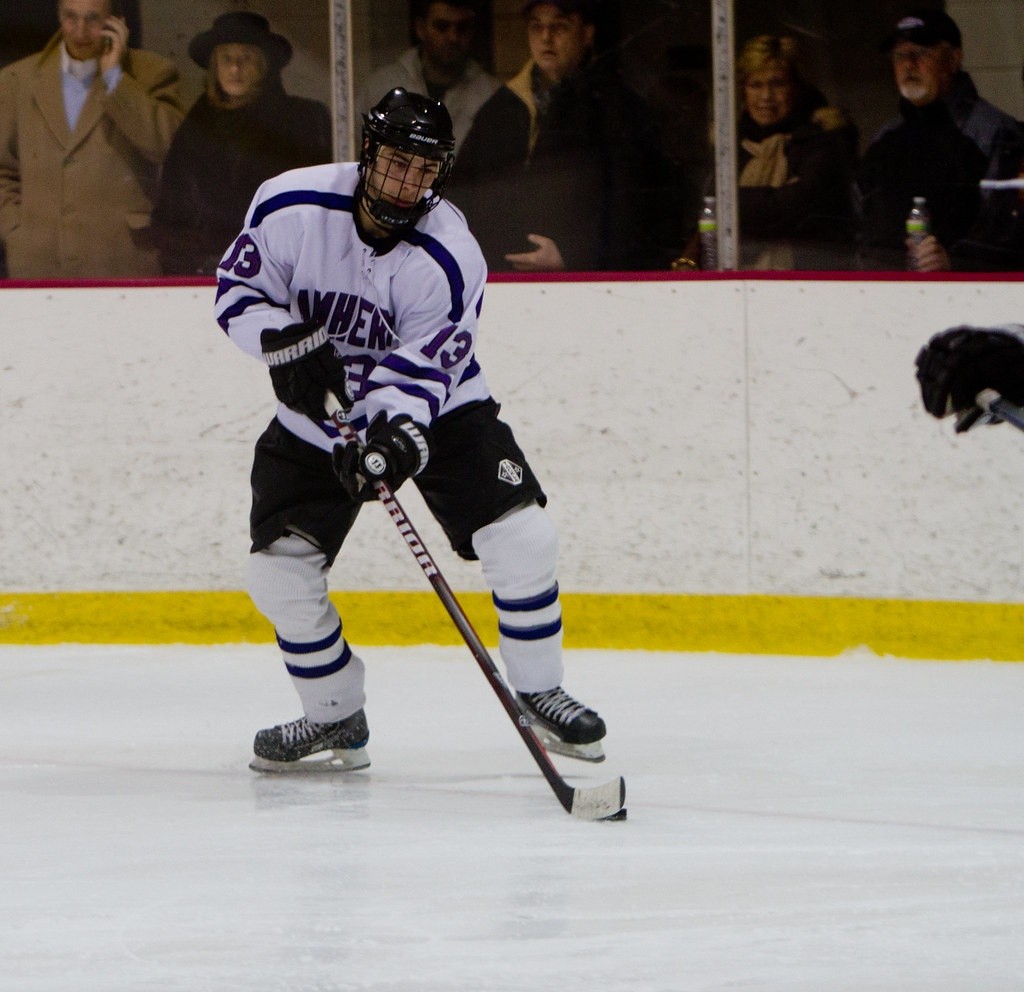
[671,255,697,267]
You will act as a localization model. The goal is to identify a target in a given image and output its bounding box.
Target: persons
[0,0,183,279]
[214,87,607,764]
[355,0,499,159]
[663,9,1023,274]
[455,0,650,273]
[148,12,333,279]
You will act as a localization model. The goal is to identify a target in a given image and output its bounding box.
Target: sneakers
[504,686,607,763]
[248,704,372,772]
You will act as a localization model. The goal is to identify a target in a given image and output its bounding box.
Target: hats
[885,9,962,49]
[186,8,293,71]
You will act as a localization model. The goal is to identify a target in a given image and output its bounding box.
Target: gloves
[259,310,354,423]
[330,408,435,504]
[916,325,1024,434]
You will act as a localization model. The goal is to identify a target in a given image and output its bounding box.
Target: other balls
[598,808,629,822]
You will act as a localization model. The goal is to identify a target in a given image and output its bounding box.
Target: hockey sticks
[322,382,630,825]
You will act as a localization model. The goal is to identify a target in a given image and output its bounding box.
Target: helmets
[358,86,457,229]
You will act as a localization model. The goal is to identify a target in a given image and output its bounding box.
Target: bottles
[699,197,717,270]
[906,196,929,271]
